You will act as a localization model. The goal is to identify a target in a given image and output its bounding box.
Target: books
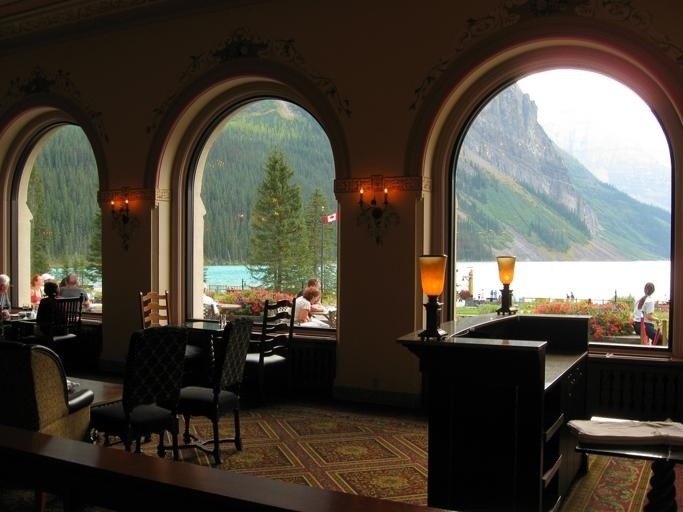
[565,418,682,452]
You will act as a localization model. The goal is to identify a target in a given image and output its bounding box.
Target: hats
[65,273,78,288]
[45,282,59,297]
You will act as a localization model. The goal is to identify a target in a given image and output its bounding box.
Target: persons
[61,271,91,310]
[202,282,220,318]
[279,285,326,324]
[35,280,68,334]
[31,273,44,304]
[632,282,666,345]
[0,273,13,323]
[292,279,321,308]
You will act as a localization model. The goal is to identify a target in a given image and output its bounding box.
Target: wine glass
[23,303,29,320]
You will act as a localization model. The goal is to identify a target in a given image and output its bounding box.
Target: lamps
[496,256,518,316]
[418,254,448,342]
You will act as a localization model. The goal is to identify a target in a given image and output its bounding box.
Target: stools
[575,416,683,511]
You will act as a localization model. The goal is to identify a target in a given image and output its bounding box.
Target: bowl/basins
[18,312,31,319]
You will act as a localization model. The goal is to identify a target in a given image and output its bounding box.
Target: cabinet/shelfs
[395,311,592,512]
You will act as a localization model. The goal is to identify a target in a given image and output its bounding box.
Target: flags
[323,213,340,224]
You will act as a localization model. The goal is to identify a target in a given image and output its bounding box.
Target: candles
[359,188,389,206]
[110,199,129,213]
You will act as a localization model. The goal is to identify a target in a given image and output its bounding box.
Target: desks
[3,318,37,341]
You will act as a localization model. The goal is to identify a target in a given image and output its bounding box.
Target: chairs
[91,292,296,465]
[51,293,83,341]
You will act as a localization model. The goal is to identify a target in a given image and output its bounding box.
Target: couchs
[1,342,94,439]
